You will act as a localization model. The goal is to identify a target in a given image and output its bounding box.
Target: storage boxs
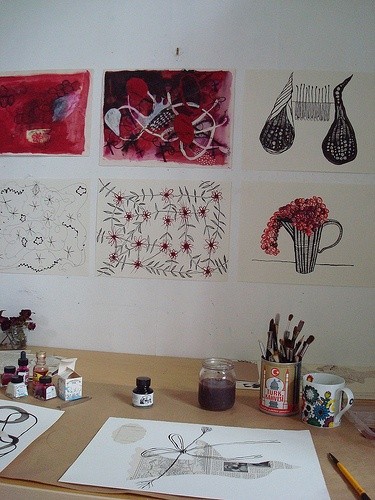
[56,357,84,402]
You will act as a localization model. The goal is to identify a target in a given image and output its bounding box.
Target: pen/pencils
[258,312,314,363]
[328,452,372,500]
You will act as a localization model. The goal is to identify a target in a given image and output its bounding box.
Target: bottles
[1,366,17,389]
[16,351,28,387]
[199,358,236,412]
[7,376,28,400]
[33,351,50,381]
[34,376,58,400]
[131,375,154,408]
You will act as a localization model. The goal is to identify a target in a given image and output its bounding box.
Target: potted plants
[0,308,39,350]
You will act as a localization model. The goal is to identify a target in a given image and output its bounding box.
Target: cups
[300,371,356,429]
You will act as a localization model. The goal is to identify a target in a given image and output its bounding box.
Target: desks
[0,343,375,500]
